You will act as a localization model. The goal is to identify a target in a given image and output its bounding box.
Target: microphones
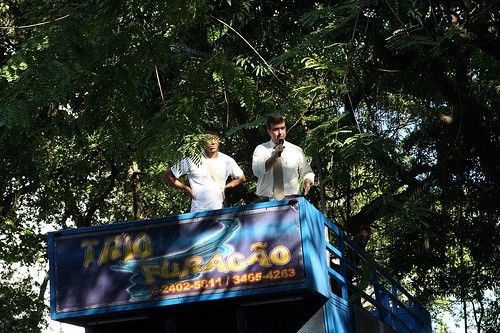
[277,138,285,157]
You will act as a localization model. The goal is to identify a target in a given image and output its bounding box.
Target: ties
[274,145,285,201]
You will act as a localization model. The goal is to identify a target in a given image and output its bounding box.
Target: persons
[252,113,315,202]
[164,127,246,213]
